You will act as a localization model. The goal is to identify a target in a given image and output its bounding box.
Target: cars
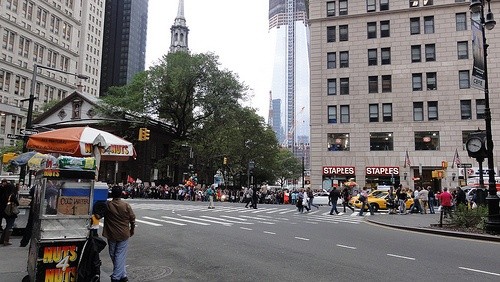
[312,192,343,205]
[353,192,416,213]
[348,190,391,207]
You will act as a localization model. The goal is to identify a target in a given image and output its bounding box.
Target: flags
[128,175,134,183]
[136,178,142,183]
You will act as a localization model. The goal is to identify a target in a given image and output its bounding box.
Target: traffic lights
[223,157,227,165]
[437,170,444,178]
[441,161,448,168]
[142,128,150,141]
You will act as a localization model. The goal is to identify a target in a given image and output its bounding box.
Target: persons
[437,187,453,219]
[245,185,257,209]
[107,182,360,205]
[329,186,341,215]
[384,184,408,215]
[98,186,136,282]
[455,186,488,207]
[359,187,374,216]
[0,179,20,245]
[296,187,319,214]
[207,184,216,208]
[408,186,439,215]
[342,188,354,213]
[19,186,35,247]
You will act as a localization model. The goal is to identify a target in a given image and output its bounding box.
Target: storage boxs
[61,181,109,207]
[75,196,90,215]
[57,196,74,216]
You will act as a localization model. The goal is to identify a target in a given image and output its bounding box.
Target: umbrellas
[8,150,56,187]
[26,125,137,161]
[344,181,358,187]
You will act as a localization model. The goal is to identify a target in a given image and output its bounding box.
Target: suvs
[463,188,484,202]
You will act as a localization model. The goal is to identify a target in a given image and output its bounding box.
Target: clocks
[467,138,482,153]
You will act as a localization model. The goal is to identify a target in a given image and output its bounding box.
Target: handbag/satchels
[5,194,20,216]
[471,201,477,209]
[358,195,366,202]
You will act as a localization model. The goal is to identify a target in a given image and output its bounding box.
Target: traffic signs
[7,134,26,141]
[20,128,39,135]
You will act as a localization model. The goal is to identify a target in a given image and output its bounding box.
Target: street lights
[19,63,90,185]
[468,0,500,235]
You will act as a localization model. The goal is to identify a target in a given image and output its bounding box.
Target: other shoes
[3,242,12,246]
[110,275,120,282]
[120,276,128,282]
[0,239,3,244]
[19,244,25,247]
